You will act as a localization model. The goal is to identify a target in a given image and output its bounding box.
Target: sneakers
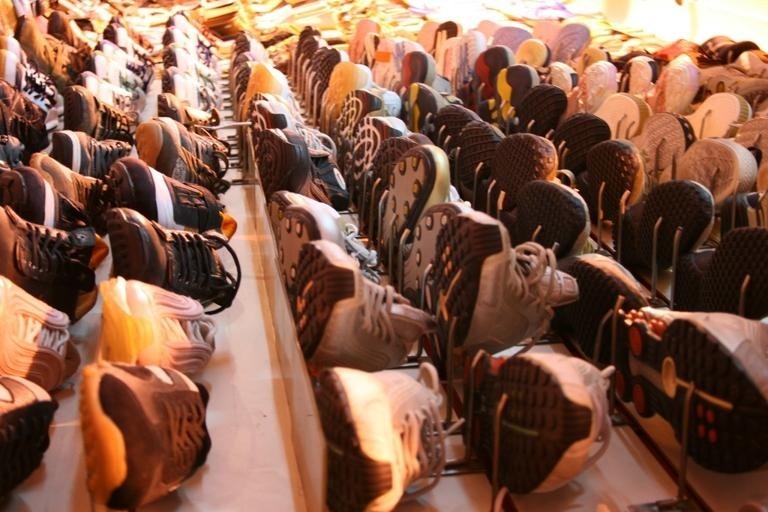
[0,374,61,496]
[287,239,442,375]
[429,207,582,378]
[0,276,82,395]
[236,19,766,317]
[98,276,218,375]
[554,252,667,369]
[308,362,468,512]
[466,349,617,496]
[2,2,242,314]
[614,303,768,476]
[77,360,213,511]
